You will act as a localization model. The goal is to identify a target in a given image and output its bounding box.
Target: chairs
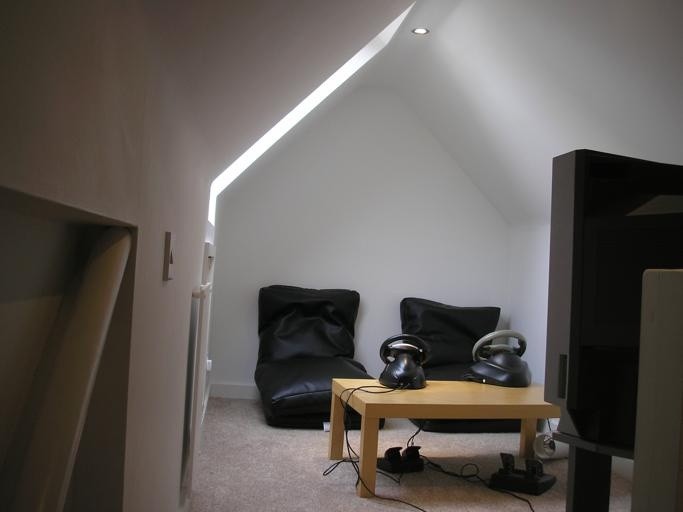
[399,296,546,432]
[255,284,386,432]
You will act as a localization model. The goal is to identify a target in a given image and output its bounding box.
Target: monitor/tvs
[543,148,683,453]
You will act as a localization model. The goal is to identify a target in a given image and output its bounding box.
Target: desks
[552,431,634,512]
